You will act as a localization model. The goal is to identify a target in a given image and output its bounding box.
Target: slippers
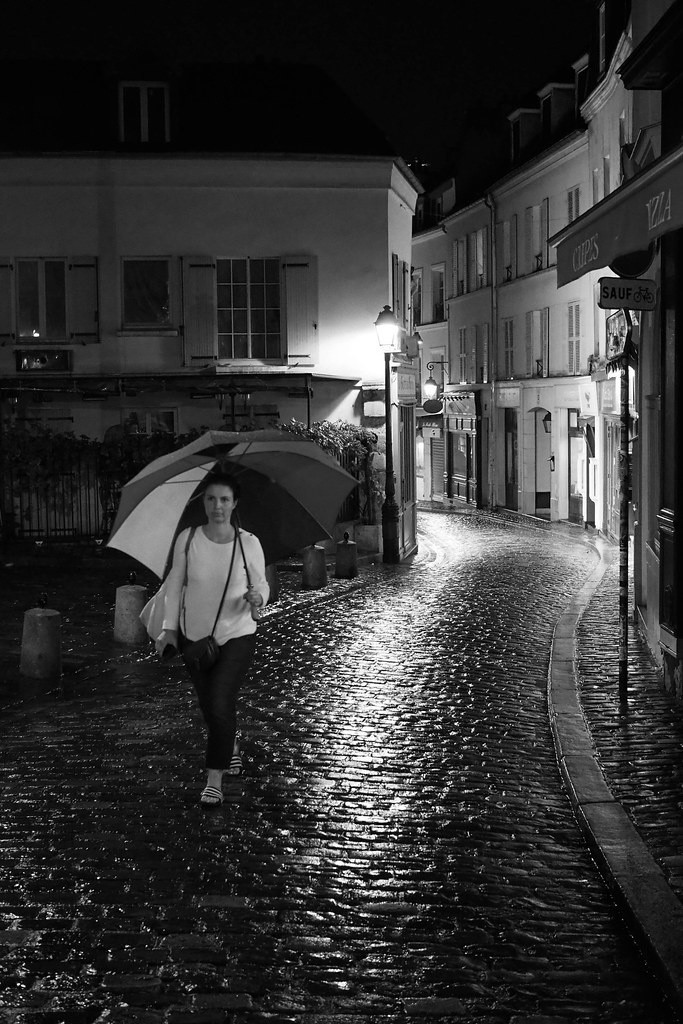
[224,740,243,777]
[199,785,225,807]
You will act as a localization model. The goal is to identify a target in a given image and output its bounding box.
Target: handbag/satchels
[178,630,221,676]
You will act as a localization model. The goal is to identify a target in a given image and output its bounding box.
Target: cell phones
[163,645,178,660]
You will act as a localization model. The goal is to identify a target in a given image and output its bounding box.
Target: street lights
[372,305,401,565]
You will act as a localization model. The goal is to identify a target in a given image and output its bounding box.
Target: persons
[140,474,270,810]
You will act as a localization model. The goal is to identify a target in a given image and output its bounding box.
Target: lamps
[424,361,449,400]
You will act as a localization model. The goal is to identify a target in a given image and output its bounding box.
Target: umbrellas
[103,426,361,590]
